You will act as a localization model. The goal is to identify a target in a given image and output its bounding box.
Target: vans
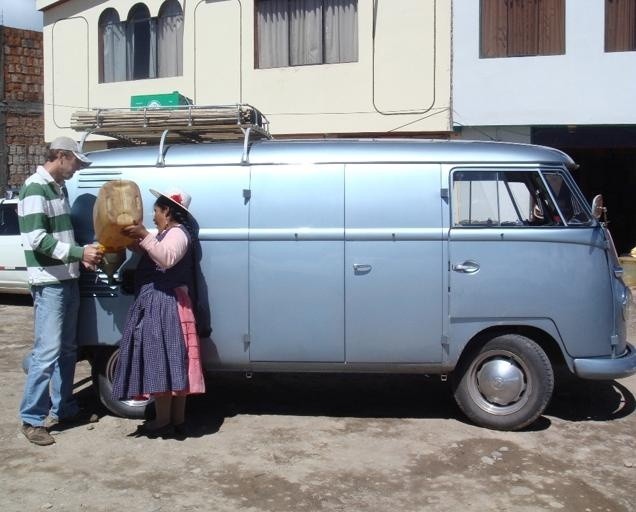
[20,116,635,435]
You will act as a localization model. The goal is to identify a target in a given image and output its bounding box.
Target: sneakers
[22,423,54,445]
[137,419,175,435]
[47,410,98,424]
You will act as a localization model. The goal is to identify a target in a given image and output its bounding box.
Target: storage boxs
[130,91,192,109]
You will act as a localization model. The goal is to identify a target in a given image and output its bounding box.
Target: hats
[149,188,199,230]
[49,137,92,169]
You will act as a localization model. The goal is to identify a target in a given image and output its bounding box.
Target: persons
[105,182,216,432]
[15,133,104,448]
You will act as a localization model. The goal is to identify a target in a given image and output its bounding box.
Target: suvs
[0,188,30,296]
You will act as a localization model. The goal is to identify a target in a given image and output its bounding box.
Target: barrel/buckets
[93,179,143,252]
